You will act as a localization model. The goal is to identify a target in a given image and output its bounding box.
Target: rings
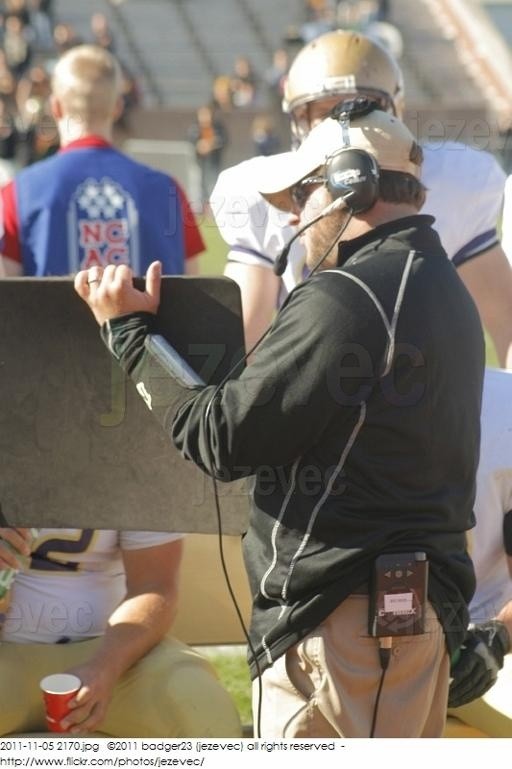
[88,277,101,285]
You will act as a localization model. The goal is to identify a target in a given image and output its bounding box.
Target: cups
[39,673,82,732]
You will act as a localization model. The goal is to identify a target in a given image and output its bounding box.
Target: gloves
[447,619,510,708]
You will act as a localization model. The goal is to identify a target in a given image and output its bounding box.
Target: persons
[0,46,204,278]
[207,32,512,366]
[0,0,403,171]
[423,371,512,736]
[73,97,484,738]
[0,527,241,737]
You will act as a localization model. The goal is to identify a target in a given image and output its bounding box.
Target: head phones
[323,96,380,214]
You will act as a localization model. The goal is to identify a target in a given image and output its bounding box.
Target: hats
[258,109,421,212]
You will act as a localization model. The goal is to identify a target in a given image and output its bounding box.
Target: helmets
[283,29,404,122]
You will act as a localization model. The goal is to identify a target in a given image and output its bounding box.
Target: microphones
[272,194,353,276]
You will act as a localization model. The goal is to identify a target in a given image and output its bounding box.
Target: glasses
[289,175,326,210]
[331,98,378,147]
